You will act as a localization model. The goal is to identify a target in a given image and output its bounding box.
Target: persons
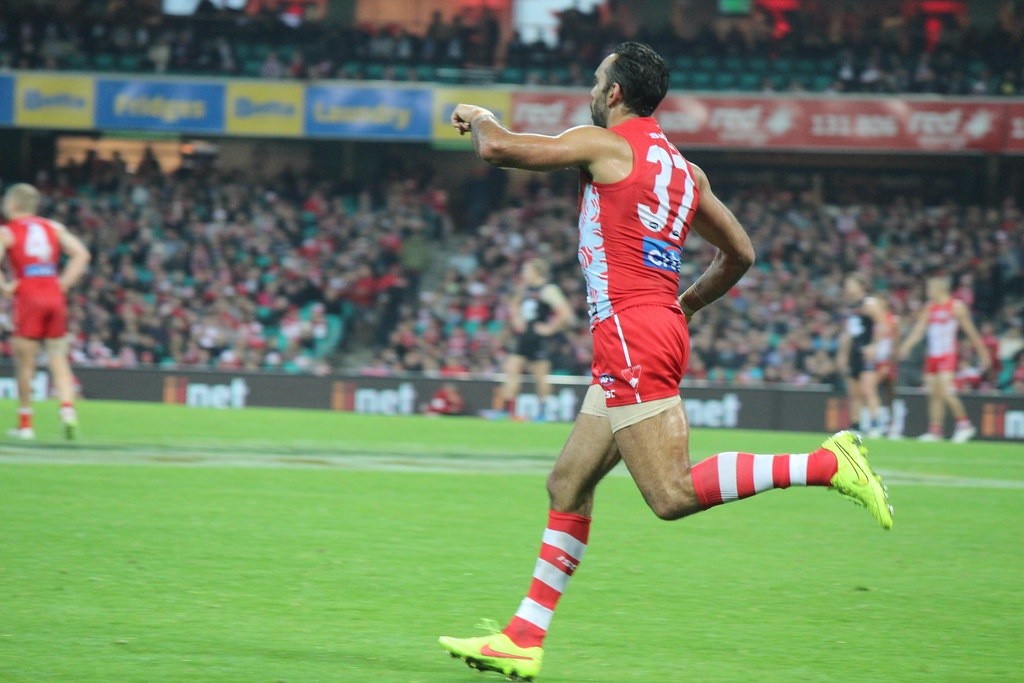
[895,275,992,445]
[0,136,1024,394]
[479,258,578,424]
[438,41,891,683]
[0,182,91,441]
[0,0,1024,97]
[838,272,899,441]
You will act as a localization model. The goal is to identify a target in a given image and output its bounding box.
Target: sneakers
[822,430,894,530]
[439,619,544,683]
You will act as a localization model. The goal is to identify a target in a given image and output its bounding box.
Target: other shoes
[9,427,34,440]
[917,433,942,442]
[952,427,976,444]
[66,417,79,441]
